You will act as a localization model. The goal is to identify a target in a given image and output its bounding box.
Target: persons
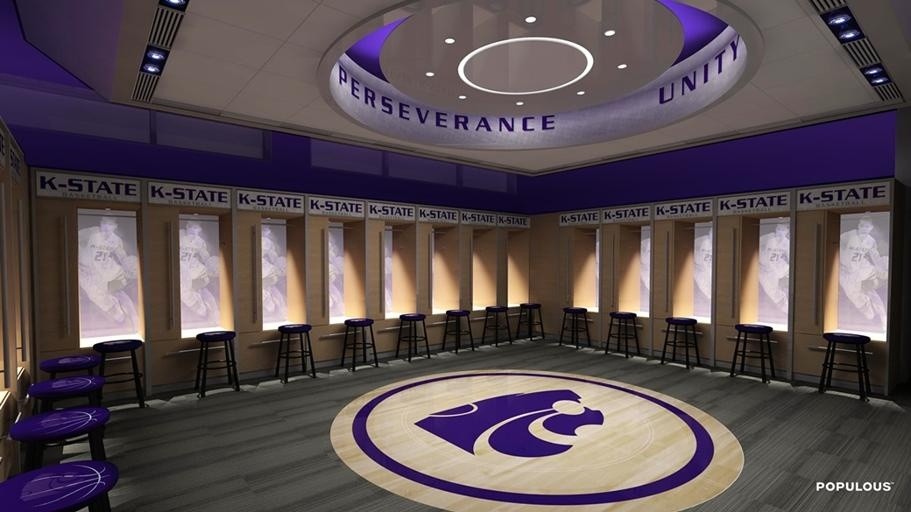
[179,217,222,323]
[78,212,140,333]
[840,216,886,333]
[640,238,651,290]
[759,223,789,315]
[694,227,712,300]
[329,231,344,311]
[262,223,288,318]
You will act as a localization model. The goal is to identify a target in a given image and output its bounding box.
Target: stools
[512,302,875,403]
[1,338,121,512]
[87,301,514,415]
